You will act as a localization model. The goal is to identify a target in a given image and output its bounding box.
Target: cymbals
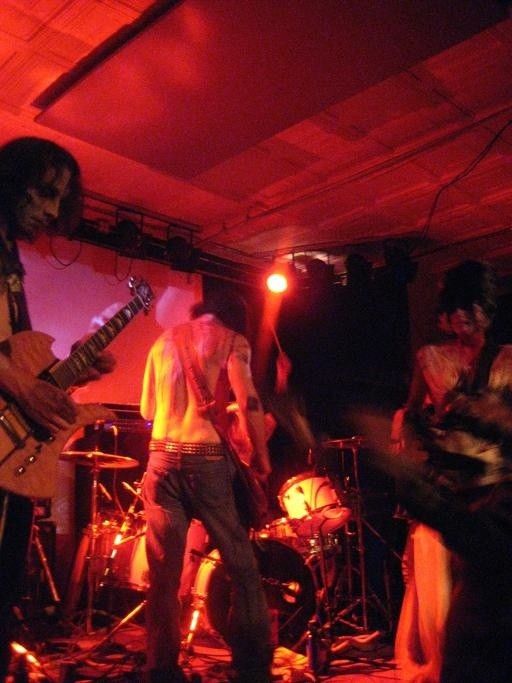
[326,430,390,449]
[58,448,139,472]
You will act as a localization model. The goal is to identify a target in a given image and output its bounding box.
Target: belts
[150,440,226,456]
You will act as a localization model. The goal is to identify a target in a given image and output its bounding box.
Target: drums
[112,512,156,592]
[278,470,347,540]
[193,533,318,656]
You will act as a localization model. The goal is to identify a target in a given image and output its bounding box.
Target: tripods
[288,451,398,670]
[65,469,144,652]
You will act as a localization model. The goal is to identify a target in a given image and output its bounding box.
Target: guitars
[0,278,155,500]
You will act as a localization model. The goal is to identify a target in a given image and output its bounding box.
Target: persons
[383,256,512,682]
[0,135,115,683]
[132,288,286,681]
